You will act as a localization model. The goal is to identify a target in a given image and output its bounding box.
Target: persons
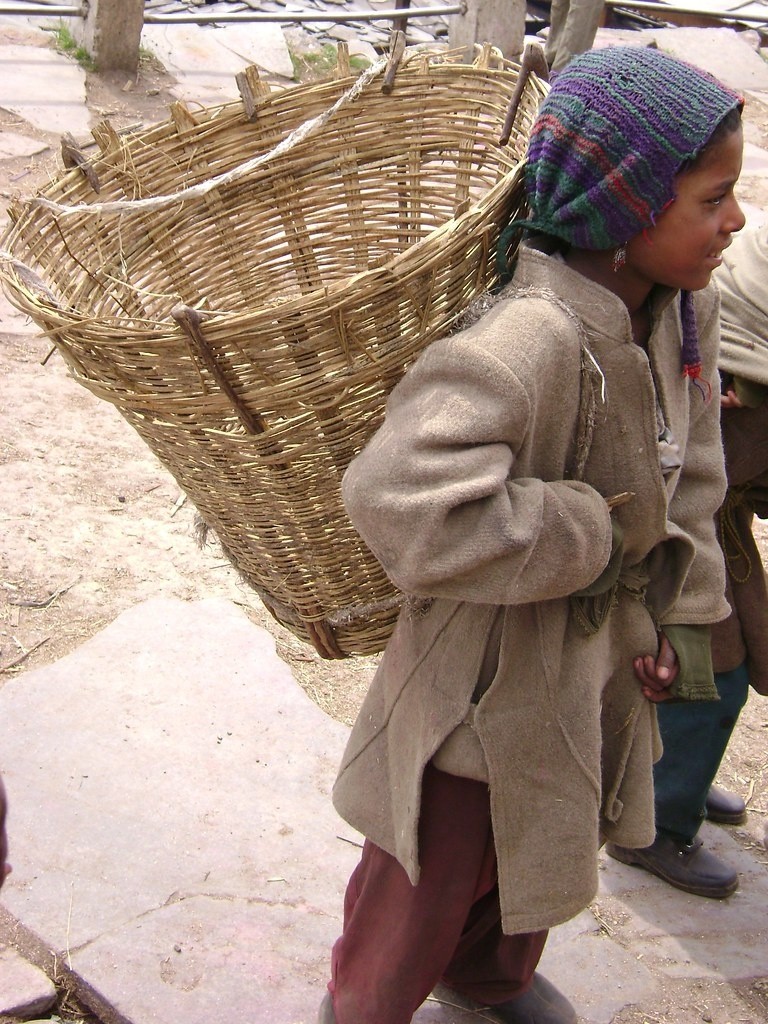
[319,47,747,1024]
[602,205,768,900]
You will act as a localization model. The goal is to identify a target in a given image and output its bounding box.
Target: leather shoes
[604,835,739,899]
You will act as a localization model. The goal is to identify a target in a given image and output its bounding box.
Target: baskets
[0,30,566,662]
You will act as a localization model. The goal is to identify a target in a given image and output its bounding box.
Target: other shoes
[318,991,336,1024]
[704,783,746,824]
[486,971,577,1024]
